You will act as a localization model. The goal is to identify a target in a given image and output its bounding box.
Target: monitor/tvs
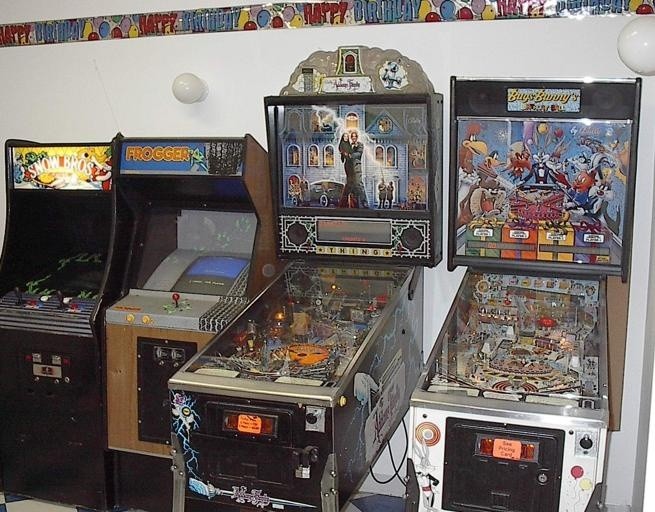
[22,249,107,298]
[170,254,250,297]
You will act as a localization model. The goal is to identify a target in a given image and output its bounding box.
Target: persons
[339,133,360,207]
[299,175,311,208]
[377,178,387,210]
[351,132,372,208]
[384,182,394,208]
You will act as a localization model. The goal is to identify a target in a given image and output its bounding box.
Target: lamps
[617,15,655,77]
[171,72,209,104]
